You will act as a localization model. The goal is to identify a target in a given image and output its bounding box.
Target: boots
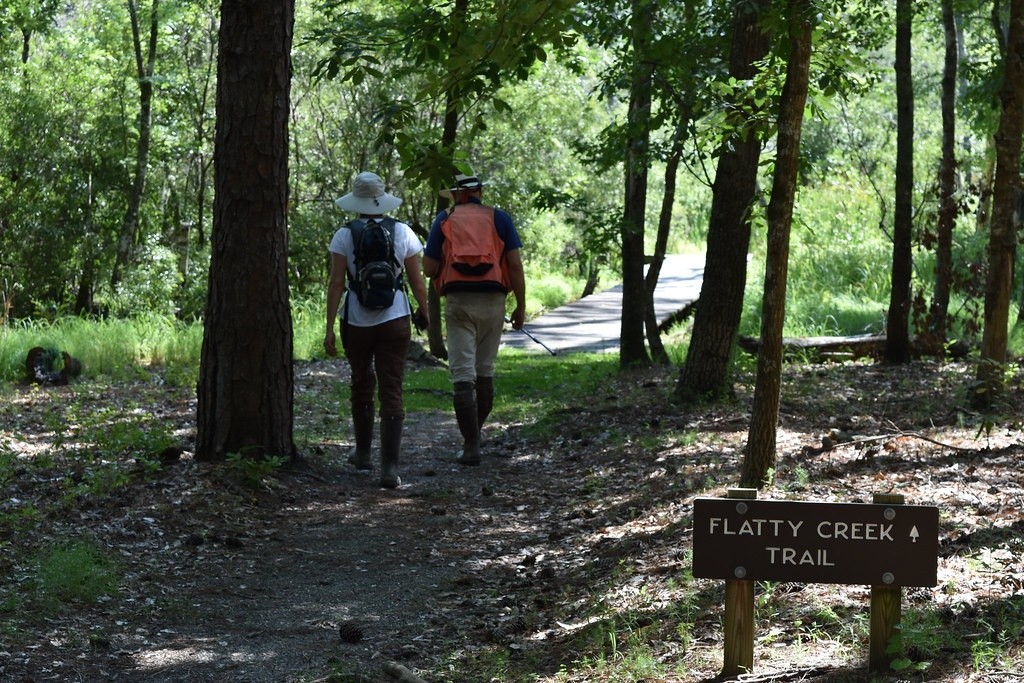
[349,414,375,469]
[380,421,401,488]
[455,405,493,466]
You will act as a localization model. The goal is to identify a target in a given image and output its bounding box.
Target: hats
[439,172,494,199]
[334,171,402,215]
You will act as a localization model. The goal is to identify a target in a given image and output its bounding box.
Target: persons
[323,172,431,487]
[423,167,525,466]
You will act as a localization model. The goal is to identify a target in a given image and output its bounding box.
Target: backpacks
[346,217,397,312]
